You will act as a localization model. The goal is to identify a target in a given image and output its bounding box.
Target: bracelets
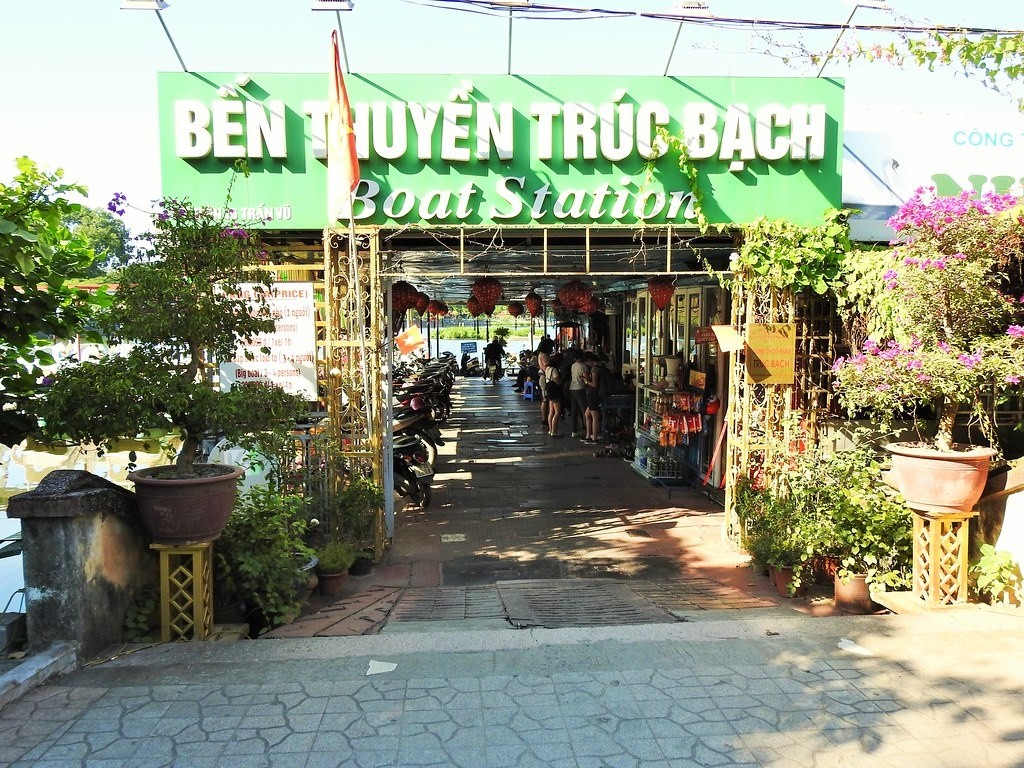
[584,379,589,385]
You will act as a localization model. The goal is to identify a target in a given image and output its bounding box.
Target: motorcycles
[310,341,483,510]
[484,347,506,384]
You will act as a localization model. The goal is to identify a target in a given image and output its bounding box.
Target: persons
[541,335,555,357]
[537,336,544,352]
[511,349,533,393]
[568,349,591,438]
[519,350,539,395]
[579,351,607,445]
[544,351,569,437]
[482,336,506,385]
[536,339,557,429]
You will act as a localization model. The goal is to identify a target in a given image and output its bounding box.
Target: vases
[129,462,241,544]
[884,440,997,515]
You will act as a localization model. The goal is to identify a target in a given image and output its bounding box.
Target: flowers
[833,178,1024,453]
[30,160,311,480]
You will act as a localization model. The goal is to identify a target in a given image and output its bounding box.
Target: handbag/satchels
[545,380,557,396]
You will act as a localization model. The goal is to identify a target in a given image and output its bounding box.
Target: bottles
[630,364,681,476]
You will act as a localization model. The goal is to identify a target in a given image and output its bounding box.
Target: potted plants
[216,422,385,630]
[731,404,912,615]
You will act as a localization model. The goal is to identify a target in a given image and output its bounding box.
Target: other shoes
[514,389,524,396]
[511,384,519,387]
[541,420,548,429]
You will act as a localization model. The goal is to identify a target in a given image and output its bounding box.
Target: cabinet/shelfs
[633,403,687,483]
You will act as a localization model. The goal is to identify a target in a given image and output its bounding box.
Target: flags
[395,324,427,356]
[710,324,746,353]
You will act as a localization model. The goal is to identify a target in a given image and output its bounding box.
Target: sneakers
[580,437,599,445]
[548,430,559,436]
[572,432,586,438]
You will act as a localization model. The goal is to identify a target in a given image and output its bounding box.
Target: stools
[523,380,540,402]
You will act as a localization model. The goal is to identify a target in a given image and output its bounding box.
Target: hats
[410,396,426,411]
